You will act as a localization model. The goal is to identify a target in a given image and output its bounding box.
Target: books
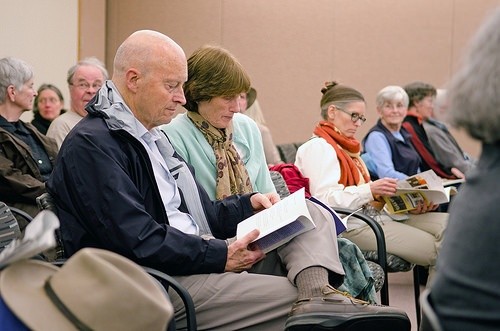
[382,170,458,214]
[236,187,316,255]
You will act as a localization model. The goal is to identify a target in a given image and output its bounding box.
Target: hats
[1,248,174,331]
[247,87,258,110]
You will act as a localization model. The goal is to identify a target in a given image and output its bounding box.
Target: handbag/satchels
[271,163,312,199]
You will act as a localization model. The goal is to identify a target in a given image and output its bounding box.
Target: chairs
[0,193,197,331]
[280,142,429,331]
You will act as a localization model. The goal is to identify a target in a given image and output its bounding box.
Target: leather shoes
[283,285,411,331]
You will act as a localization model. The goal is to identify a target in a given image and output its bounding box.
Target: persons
[431,13,500,331]
[295,81,451,288]
[425,89,471,172]
[47,58,108,150]
[239,87,277,168]
[52,31,411,331]
[0,56,64,259]
[362,86,449,210]
[400,81,463,180]
[32,84,68,136]
[160,46,376,304]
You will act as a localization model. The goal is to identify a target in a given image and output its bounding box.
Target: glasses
[335,107,366,125]
[71,83,102,90]
